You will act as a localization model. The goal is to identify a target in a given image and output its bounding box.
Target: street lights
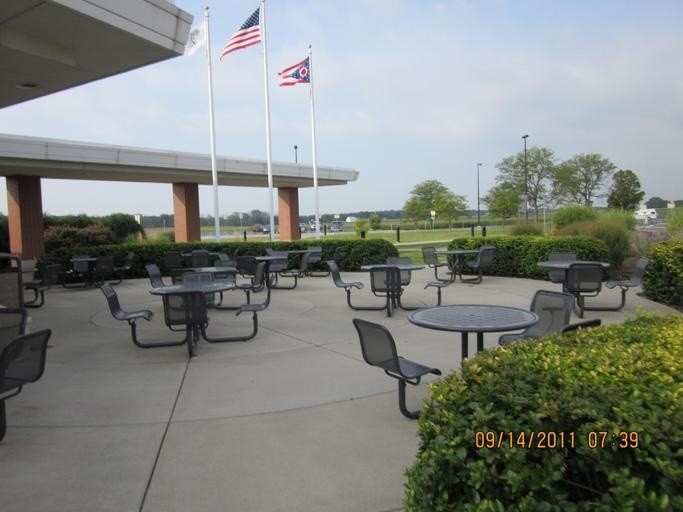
[478,162,483,226]
[521,133,530,223]
[293,143,299,163]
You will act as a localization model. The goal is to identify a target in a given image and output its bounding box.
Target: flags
[278,56,311,86]
[184,17,205,56]
[219,5,261,58]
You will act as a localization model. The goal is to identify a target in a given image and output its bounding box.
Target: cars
[252,222,342,234]
